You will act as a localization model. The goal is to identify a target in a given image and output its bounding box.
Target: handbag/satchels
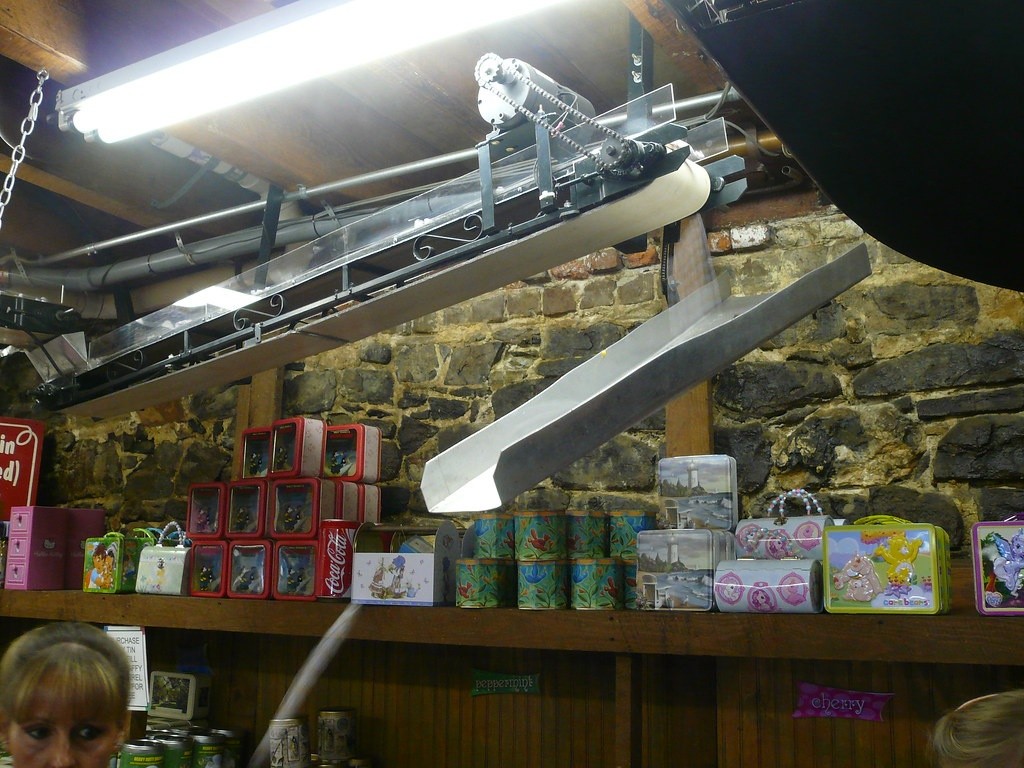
[135,521,192,595]
[713,528,823,613]
[734,488,848,560]
[971,512,1024,617]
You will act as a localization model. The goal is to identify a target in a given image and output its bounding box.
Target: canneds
[269,709,369,768]
[318,519,362,597]
[108,725,240,768]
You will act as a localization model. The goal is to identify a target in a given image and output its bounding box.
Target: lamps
[56,0,564,146]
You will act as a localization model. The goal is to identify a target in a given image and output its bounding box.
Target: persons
[0,622,131,768]
[927,690,1024,768]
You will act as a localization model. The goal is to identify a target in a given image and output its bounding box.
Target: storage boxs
[273,539,318,602]
[351,523,474,607]
[186,483,226,539]
[147,670,211,720]
[635,455,738,613]
[83,538,179,594]
[270,478,334,539]
[227,539,272,600]
[358,483,381,523]
[321,423,382,485]
[267,417,326,478]
[66,509,104,590]
[225,482,273,539]
[334,481,358,521]
[5,506,69,589]
[189,540,229,599]
[239,426,275,481]
